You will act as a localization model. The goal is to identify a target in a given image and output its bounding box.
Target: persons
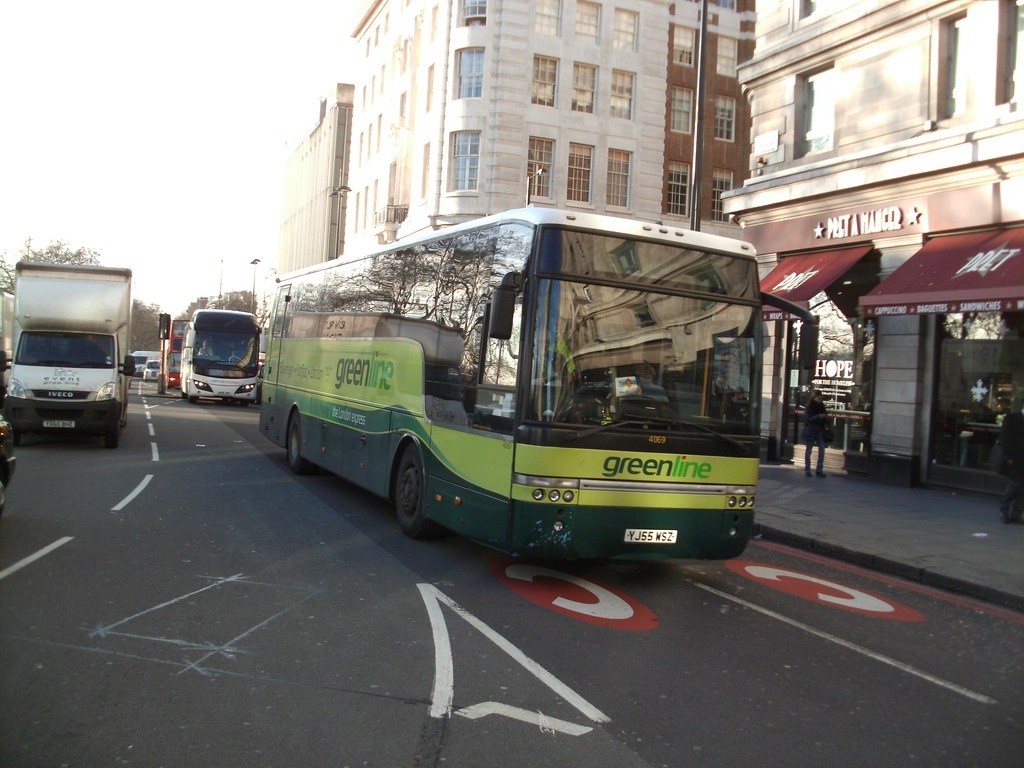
[198,339,214,356]
[710,374,750,421]
[999,414,1024,524]
[802,390,831,478]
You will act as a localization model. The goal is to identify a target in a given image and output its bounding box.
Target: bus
[259,205,820,563]
[158,319,190,390]
[180,309,266,406]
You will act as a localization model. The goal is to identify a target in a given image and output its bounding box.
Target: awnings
[858,224,1024,317]
[752,245,875,321]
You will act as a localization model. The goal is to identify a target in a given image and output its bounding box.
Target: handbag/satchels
[824,424,834,442]
[990,444,1006,476]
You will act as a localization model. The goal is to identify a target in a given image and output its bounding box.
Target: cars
[142,359,160,382]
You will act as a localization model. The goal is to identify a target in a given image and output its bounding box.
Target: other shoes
[806,471,811,476]
[817,471,826,477]
[1002,516,1009,523]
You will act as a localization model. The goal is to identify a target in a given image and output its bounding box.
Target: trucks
[2,260,134,450]
[131,350,160,377]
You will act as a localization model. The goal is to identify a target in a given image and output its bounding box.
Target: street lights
[526,168,547,207]
[250,259,261,313]
[329,186,353,257]
